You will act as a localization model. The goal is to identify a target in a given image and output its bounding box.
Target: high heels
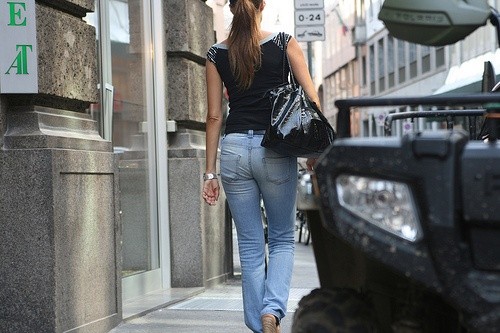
[260,313,282,333]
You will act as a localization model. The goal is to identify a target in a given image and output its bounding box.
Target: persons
[201,0,321,333]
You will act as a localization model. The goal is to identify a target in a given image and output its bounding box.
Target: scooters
[287,0,500,333]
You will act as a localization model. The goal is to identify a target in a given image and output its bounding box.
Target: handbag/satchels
[260,83,336,157]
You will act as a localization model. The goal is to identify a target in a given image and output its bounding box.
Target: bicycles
[295,161,312,246]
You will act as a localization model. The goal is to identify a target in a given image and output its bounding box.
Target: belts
[229,129,267,135]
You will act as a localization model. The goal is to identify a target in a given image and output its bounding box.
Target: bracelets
[203,171,219,180]
